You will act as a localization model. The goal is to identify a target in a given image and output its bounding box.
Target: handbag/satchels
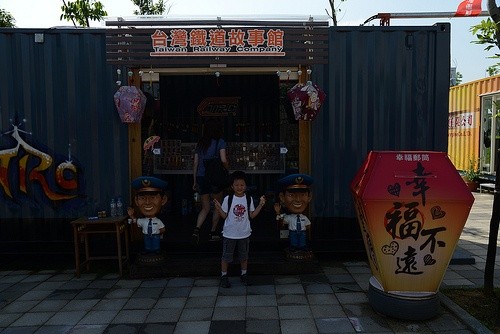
[205,137,231,187]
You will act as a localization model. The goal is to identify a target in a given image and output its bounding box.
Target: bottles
[110,199,117,217]
[182,187,215,216]
[102,209,106,217]
[117,197,123,217]
[98,210,103,218]
[286,144,299,169]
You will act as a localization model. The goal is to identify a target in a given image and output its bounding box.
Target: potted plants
[460,165,480,191]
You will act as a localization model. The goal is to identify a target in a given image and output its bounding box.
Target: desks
[71,214,129,278]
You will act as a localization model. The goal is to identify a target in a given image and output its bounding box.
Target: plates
[88,216,98,220]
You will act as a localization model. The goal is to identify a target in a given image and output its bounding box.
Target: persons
[274,172,314,252]
[213,170,267,288]
[127,176,169,258]
[192,118,228,242]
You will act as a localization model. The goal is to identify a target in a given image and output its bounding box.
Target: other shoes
[191,226,201,237]
[240,273,251,286]
[220,275,231,288]
[207,232,223,241]
